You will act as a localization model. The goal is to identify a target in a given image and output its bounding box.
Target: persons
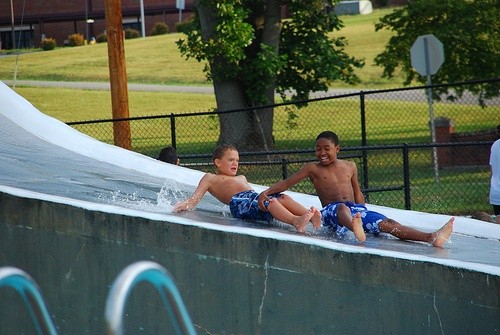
[171,144,321,233]
[159,144,177,166]
[472,124,500,224]
[258,131,455,248]
